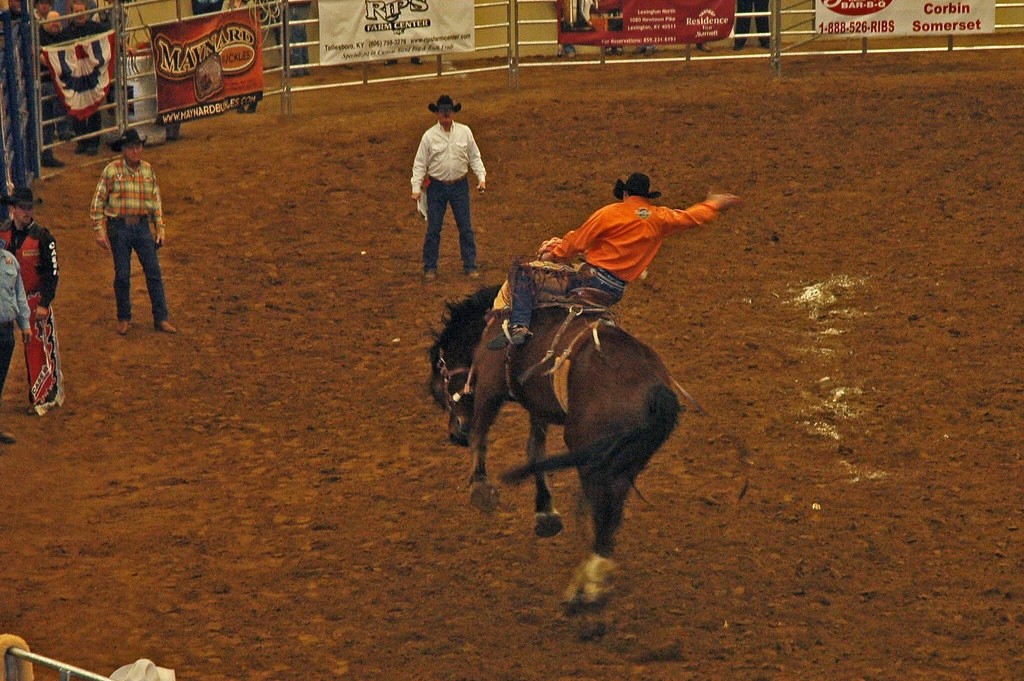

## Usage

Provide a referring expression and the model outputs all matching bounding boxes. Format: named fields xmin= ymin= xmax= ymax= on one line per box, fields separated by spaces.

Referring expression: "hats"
xmin=610 ymin=172 xmax=661 ymax=200
xmin=32 ymin=0 xmax=53 ymax=8
xmin=428 ymin=92 xmax=464 ymax=113
xmin=1 ymin=185 xmax=42 ymax=211
xmin=109 ymin=128 xmax=148 ymax=152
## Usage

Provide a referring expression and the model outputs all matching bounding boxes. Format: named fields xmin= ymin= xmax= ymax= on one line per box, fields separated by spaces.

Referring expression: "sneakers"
xmin=117 ymin=318 xmax=130 ymax=334
xmin=154 ymin=319 xmax=177 ymax=333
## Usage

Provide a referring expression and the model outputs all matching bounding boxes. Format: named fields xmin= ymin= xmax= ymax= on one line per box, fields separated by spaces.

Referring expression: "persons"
xmin=384 ymin=55 xmax=423 ymax=67
xmin=1 ymin=0 xmax=41 ymax=126
xmin=90 ymin=127 xmax=178 ymax=335
xmin=605 ymin=45 xmax=624 ymax=57
xmin=564 ymin=44 xmax=577 ymax=58
xmin=410 ymin=94 xmax=487 ymax=282
xmin=28 ymin=0 xmax=69 ymax=143
xmin=286 ymin=0 xmax=311 ymax=78
xmin=269 ymin=0 xmax=288 ymax=76
xmin=343 ymin=64 xmax=354 ymax=69
xmin=61 ymin=2 xmax=109 ymax=158
xmin=0 ymin=185 xmax=60 ymax=416
xmin=732 ymin=0 xmax=772 ymax=50
xmin=691 ymin=43 xmax=711 ymax=52
xmin=99 ymin=1 xmax=137 ymax=114
xmin=0 ymin=239 xmax=34 ymax=444
xmin=485 ymin=172 xmax=743 ymax=356
xmin=631 ymin=45 xmax=658 ymax=58
xmin=33 ymin=11 xmax=77 ymax=168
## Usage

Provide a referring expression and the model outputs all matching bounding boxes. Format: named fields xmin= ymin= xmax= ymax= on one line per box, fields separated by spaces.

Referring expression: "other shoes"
xmin=0 ymin=429 xmax=17 ymax=445
xmin=75 ymin=139 xmax=87 ymax=155
xmin=732 ymin=40 xmax=772 ymax=52
xmin=424 ymin=266 xmax=438 ymax=282
xmin=602 ymin=45 xmax=627 ymax=57
xmin=285 ymin=66 xmax=311 ymax=79
xmin=382 ymin=56 xmax=400 ymax=67
xmin=694 ymin=38 xmax=713 ymax=51
xmin=84 ymin=142 xmax=101 ymax=155
xmin=463 ymin=266 xmax=482 ymax=278
xmin=631 ymin=41 xmax=658 ymax=56
xmin=410 ymin=56 xmax=425 ymax=66
xmin=487 ymin=327 xmax=529 ymax=352
xmin=40 ymin=157 xmax=66 ymax=167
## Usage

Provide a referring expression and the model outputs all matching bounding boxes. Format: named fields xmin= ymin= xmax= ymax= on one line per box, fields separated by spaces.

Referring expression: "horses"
xmin=426 ymin=285 xmax=681 ymax=615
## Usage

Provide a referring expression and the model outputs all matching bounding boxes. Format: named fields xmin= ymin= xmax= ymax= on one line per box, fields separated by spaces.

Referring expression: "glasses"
xmin=437 ymin=108 xmax=455 ymax=114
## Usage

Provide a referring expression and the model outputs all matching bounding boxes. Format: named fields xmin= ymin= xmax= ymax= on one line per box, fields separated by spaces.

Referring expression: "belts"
xmin=434 ymin=176 xmax=467 ymax=185
xmin=110 ymin=215 xmax=153 ymax=227
